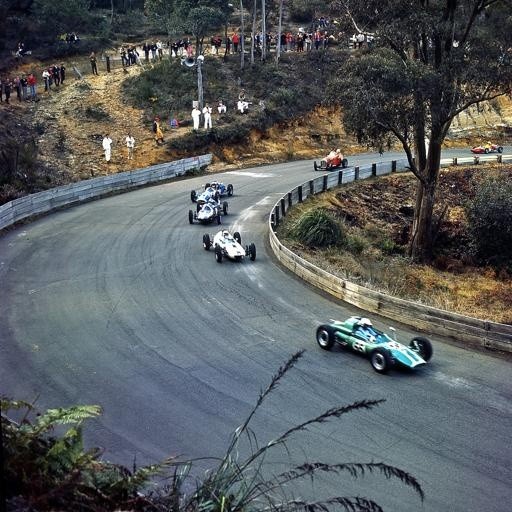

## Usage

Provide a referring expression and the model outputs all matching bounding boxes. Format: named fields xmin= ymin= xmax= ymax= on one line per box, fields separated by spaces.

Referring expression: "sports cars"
xmin=315 ymin=314 xmax=432 ymax=375
xmin=469 ymin=142 xmax=503 ymax=154
xmin=312 ymin=150 xmax=348 ymax=172
xmin=188 ymin=181 xmax=256 ymax=264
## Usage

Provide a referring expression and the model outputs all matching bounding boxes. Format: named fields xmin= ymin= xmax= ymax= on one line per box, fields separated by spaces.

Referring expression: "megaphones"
xmin=185 ymin=57 xmax=196 ymax=67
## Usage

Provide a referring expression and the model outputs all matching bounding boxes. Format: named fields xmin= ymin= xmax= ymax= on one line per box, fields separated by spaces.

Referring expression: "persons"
xmin=202 ymin=102 xmax=213 ymax=129
xmin=143 ymin=39 xmax=163 ymax=59
xmin=219 ymin=231 xmax=234 ymax=244
xmin=216 ymin=97 xmax=227 ymax=114
xmin=211 ymin=183 xmax=216 ymax=190
xmin=485 ymin=141 xmax=491 ymax=149
xmin=168 ymin=37 xmax=193 ymax=57
xmin=152 ymin=117 xmax=165 ymax=147
xmin=238 ymin=89 xmax=249 ymax=113
xmin=125 ymin=132 xmax=136 ymax=160
xmin=89 ymin=51 xmax=98 ymax=75
xmin=352 ymin=31 xmax=374 ymax=49
xmin=102 ymin=133 xmax=113 ymax=164
xmin=255 ymin=30 xmax=329 ymax=53
xmin=43 ymin=62 xmax=66 ymax=92
xmin=120 ymin=45 xmax=139 ymax=66
xmin=357 ymin=318 xmax=381 ymax=343
xmin=211 ymin=32 xmax=242 ymax=55
xmin=318 ymin=16 xmax=328 ymax=28
xmin=66 ymin=31 xmax=80 ymax=44
xmin=190 ymin=105 xmax=201 ymax=131
xmin=4 ymin=72 xmax=37 ymax=103
xmin=105 ymin=51 xmax=111 ymax=72
